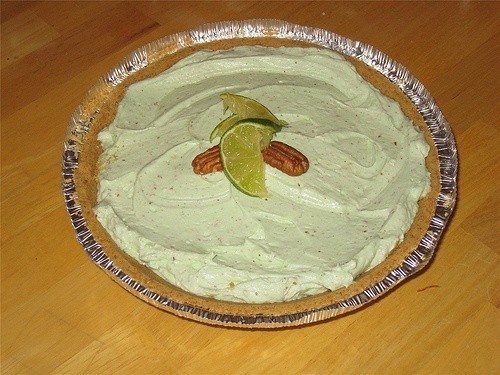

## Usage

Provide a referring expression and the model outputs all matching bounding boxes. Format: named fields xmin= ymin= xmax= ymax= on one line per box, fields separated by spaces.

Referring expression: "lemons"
xmin=210 ymin=93 xmax=289 ymax=199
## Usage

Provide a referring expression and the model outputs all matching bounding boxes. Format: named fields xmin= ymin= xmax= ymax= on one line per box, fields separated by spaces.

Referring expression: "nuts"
xmin=262 ymin=141 xmax=309 ymax=176
xmin=191 ymin=143 xmax=222 ymax=175
